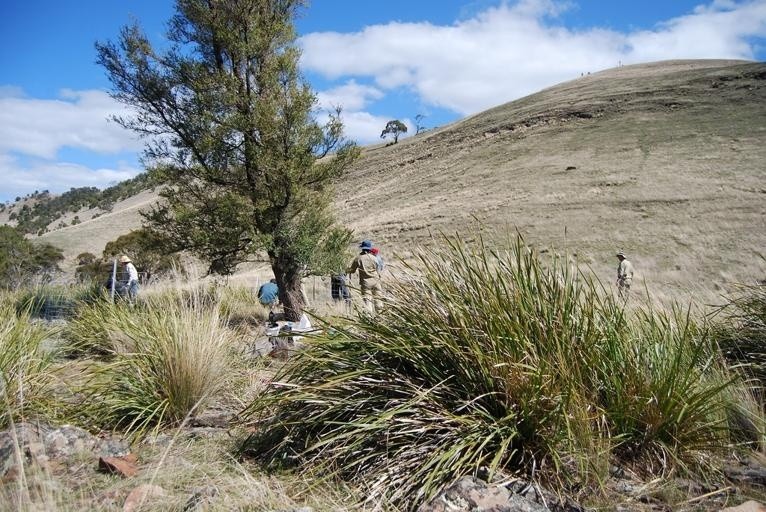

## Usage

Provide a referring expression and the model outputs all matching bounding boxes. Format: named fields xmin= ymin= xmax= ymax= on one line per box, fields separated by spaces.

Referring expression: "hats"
xmin=370 ymin=249 xmax=378 ymax=255
xmin=118 ymin=255 xmax=133 ymax=263
xmin=616 ymin=251 xmax=626 ymax=259
xmin=359 ymin=239 xmax=373 ymax=250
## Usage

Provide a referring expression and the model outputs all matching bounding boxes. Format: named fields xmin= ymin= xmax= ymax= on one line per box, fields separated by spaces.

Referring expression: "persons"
xmin=330 ymin=271 xmax=350 ymax=304
xmin=105 ymin=270 xmax=125 ymax=304
xmin=615 ymin=250 xmax=634 ymax=302
xmin=257 ymin=278 xmax=283 ymax=309
xmin=347 ymin=239 xmax=383 ymax=313
xmin=296 ymin=278 xmax=310 ymax=307
xmin=370 ymin=248 xmax=384 ymax=272
xmin=118 ymin=254 xmax=138 ymax=303
